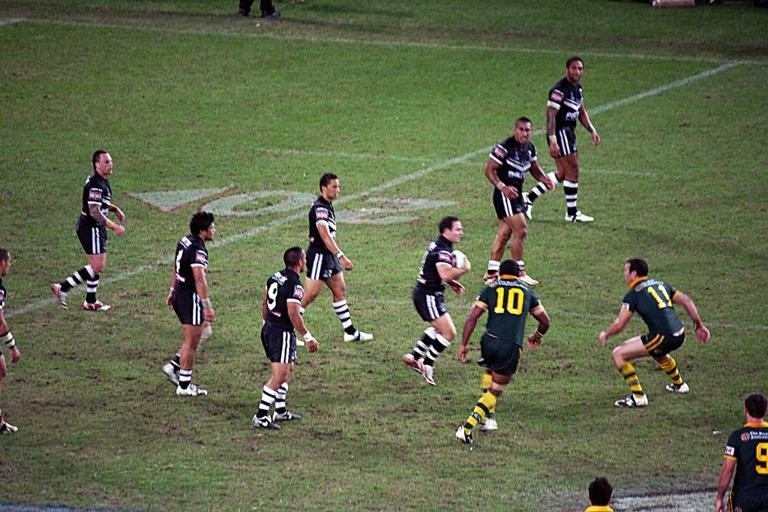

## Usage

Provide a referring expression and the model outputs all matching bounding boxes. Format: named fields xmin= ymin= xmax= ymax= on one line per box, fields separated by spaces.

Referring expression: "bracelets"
xmin=303 ymin=331 xmax=314 ymax=343
xmin=0 ymin=331 xmax=16 ymax=349
xmin=202 ymin=298 xmax=212 ymax=309
xmin=168 ymin=286 xmax=176 ymax=294
xmin=548 ymin=135 xmax=557 ymax=145
xmin=106 ymin=220 xmax=116 ymax=230
xmin=497 ymin=181 xmax=505 ymax=192
xmin=334 ymin=250 xmax=343 ymax=258
xmin=533 ymin=329 xmax=543 ymax=339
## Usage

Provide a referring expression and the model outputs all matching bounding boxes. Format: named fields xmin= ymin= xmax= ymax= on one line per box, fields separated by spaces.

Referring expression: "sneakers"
xmin=0 ymin=415 xmax=19 ymax=434
xmin=51 ymin=283 xmax=68 ymax=310
xmin=522 ymin=192 xmax=534 ymax=220
xmin=273 ymin=411 xmax=301 ymax=421
xmin=666 ymin=382 xmax=689 ymax=394
xmin=456 ymin=418 xmax=498 ymax=443
xmin=565 ymin=210 xmax=594 ymax=222
xmin=83 ymin=300 xmax=111 ymax=311
xmin=162 ymin=362 xmax=207 ymax=396
xmin=344 ymin=330 xmax=373 ymax=341
xmin=483 ymin=269 xmax=539 ymax=286
xmin=614 ymin=394 xmax=648 ymax=406
xmin=252 ymin=414 xmax=281 ymax=430
xmin=403 ymin=354 xmax=437 ymax=386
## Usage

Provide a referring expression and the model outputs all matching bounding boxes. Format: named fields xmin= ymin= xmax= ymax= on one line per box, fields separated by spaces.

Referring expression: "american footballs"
xmin=450 ymin=249 xmax=466 ymax=269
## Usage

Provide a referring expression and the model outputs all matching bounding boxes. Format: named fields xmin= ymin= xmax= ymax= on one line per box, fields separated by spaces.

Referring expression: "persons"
xmin=161 ymin=211 xmax=216 ymax=396
xmin=1 ymin=247 xmax=21 ymax=434
xmin=252 ymin=247 xmax=319 ymax=430
xmin=52 ymin=151 xmax=126 ymax=311
xmin=455 ymin=258 xmax=550 ymax=443
xmin=403 ymin=216 xmax=472 ymax=386
xmin=600 ymin=259 xmax=713 ymax=407
xmin=299 ymin=173 xmax=372 ymax=343
xmin=716 ymin=392 xmax=768 ymax=512
xmin=584 ymin=477 xmax=614 ymax=512
xmin=482 ymin=118 xmax=555 ymax=285
xmin=521 ymin=57 xmax=600 ymax=222
xmin=240 ymin=1 xmax=280 ymax=20
